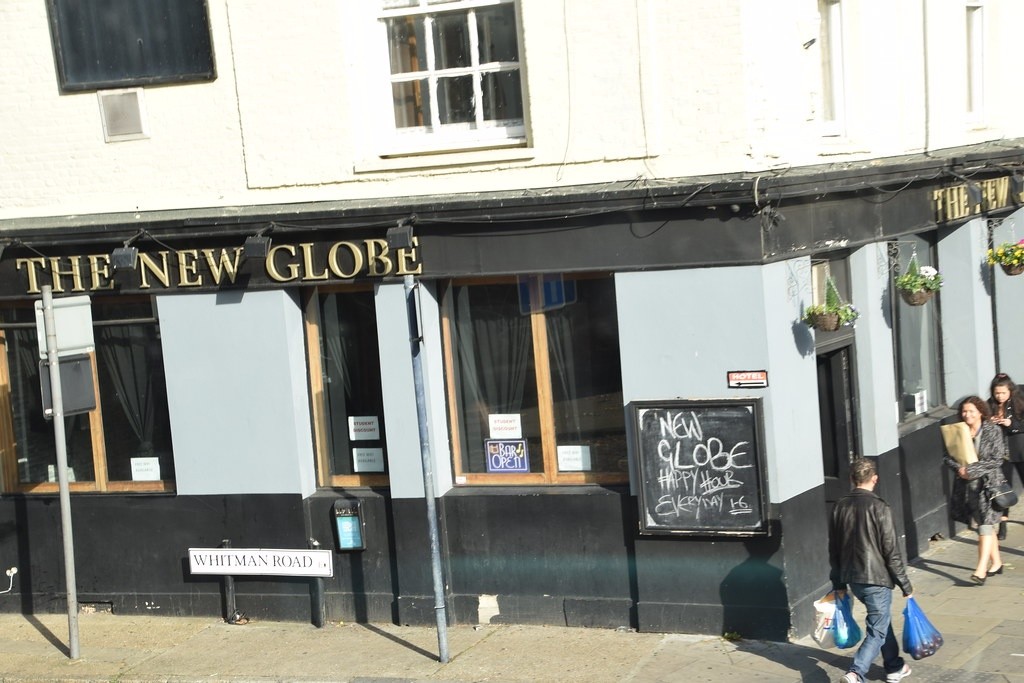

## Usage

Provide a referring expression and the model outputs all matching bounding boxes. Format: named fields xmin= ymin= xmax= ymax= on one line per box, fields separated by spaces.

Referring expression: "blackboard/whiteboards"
xmin=629 ymin=396 xmax=773 ymax=538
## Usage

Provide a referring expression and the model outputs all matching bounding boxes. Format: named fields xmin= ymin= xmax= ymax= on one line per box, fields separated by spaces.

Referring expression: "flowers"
xmin=803 ymin=304 xmax=861 ymax=330
xmin=986 ymin=239 xmax=1024 ymax=272
xmin=896 ymin=259 xmax=945 ymax=296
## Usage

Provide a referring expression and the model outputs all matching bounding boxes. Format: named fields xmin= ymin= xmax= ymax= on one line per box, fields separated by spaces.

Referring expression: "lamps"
xmin=987 ymin=162 xmax=1024 ymax=194
xmin=387 ymin=214 xmax=419 ymax=250
xmin=942 ymin=168 xmax=984 ymax=209
xmin=111 ymin=228 xmax=146 ymax=271
xmin=243 ymin=222 xmax=277 ymax=257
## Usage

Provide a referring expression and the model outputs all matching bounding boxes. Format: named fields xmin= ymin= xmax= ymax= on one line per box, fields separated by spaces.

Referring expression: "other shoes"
xmin=970 ymin=573 xmax=987 ymax=585
xmin=986 ymin=565 xmax=1004 ymax=577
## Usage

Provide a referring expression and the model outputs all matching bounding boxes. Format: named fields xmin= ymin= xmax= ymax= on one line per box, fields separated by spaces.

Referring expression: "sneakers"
xmin=840 ymin=671 xmax=860 ymax=683
xmin=886 ymin=664 xmax=912 ymax=683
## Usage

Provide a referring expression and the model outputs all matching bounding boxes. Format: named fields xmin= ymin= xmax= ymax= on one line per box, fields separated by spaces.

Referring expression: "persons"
xmin=829 ymin=458 xmax=914 ymax=683
xmin=986 ymin=373 xmax=1024 ymax=540
xmin=943 ymin=395 xmax=1007 ymax=586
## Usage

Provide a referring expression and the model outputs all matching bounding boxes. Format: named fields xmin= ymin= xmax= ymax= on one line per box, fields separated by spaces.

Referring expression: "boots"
xmin=996 ymin=516 xmax=1008 ymax=540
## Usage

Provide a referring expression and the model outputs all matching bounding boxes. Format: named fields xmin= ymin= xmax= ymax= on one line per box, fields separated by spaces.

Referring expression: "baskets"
xmin=899 ymin=288 xmax=934 ymax=306
xmin=814 ymin=311 xmax=841 ymax=331
xmin=999 ymin=261 xmax=1024 ymax=276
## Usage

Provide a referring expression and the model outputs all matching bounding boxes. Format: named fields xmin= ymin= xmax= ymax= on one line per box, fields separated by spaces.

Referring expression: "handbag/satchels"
xmin=984 ymin=479 xmax=1019 ymax=513
xmin=903 ymin=597 xmax=944 ymax=660
xmin=811 ymin=588 xmax=862 ymax=649
